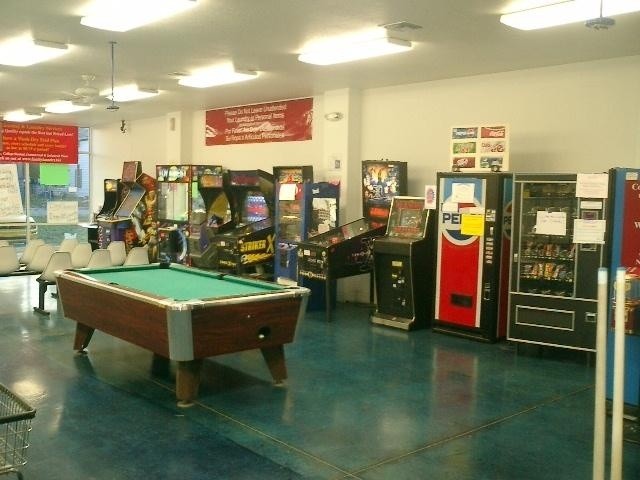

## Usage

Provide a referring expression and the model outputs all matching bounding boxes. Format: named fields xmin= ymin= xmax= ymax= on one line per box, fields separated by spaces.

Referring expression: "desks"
xmin=54 ymin=262 xmax=312 ymax=408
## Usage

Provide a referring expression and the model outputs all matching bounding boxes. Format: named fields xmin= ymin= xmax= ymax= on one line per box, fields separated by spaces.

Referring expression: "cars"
xmin=0 ymin=215 xmax=38 ymax=244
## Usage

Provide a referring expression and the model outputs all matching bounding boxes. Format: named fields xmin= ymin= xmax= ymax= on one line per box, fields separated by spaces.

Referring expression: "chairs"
xmin=0 ymin=238 xmax=149 ymax=316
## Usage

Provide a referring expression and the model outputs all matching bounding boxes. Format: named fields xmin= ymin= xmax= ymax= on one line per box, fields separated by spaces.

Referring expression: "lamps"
xmin=500 ymin=1 xmax=640 ymax=31
xmin=80 ymin=0 xmax=197 ymax=33
xmin=169 ymin=60 xmax=257 ymax=88
xmin=0 ymin=37 xmax=68 ymax=67
xmin=296 ymin=21 xmax=422 ymax=66
xmin=5 ymin=82 xmax=159 ymax=123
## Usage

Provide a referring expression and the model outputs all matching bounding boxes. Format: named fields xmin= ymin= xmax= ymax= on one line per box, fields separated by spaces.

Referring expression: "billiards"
xmin=258 ymin=334 xmax=264 ymax=339
